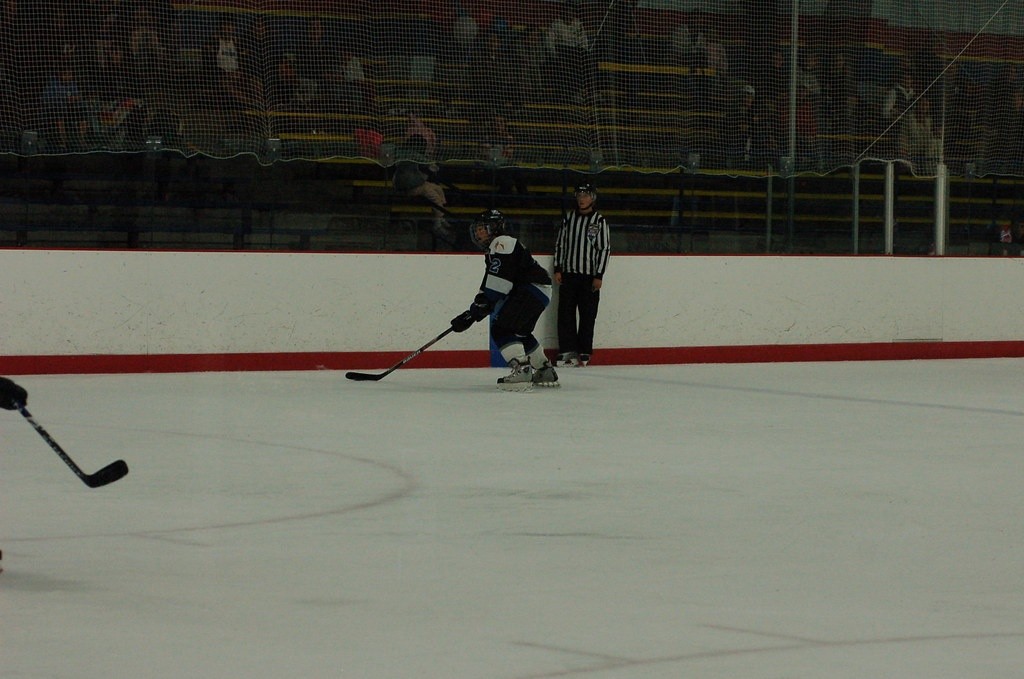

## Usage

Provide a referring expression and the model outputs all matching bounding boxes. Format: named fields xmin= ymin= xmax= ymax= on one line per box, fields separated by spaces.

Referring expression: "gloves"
xmin=450 ymin=309 xmax=477 ymax=333
xmin=0 ymin=377 xmax=27 ymax=411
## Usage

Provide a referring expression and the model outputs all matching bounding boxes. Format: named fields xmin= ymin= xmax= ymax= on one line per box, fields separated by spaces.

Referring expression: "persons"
xmin=554 ymin=180 xmax=610 ymax=367
xmin=0 ymin=3 xmax=980 ymax=227
xmin=1 ymin=376 xmax=27 ymax=410
xmin=451 ymin=210 xmax=562 ymax=393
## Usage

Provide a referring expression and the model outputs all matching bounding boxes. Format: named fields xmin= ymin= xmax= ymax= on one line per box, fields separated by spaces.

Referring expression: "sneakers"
xmin=579 ymin=353 xmax=592 ymax=368
xmin=494 ymin=356 xmax=536 ymax=393
xmin=531 ymin=361 xmax=559 ymax=388
xmin=550 ymin=351 xmax=580 ymax=368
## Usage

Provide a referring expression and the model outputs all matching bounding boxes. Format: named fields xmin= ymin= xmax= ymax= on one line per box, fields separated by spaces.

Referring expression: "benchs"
xmin=172 ymin=55 xmax=1024 ymax=245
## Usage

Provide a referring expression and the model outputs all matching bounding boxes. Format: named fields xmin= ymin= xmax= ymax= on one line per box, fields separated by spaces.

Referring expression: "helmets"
xmin=474 ymin=208 xmax=513 ymax=236
xmin=575 ymin=177 xmax=598 ymax=195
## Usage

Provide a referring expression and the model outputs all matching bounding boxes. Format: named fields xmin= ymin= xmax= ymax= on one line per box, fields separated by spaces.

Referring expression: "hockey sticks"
xmin=345 ymin=327 xmax=454 ymax=381
xmin=12 ymin=398 xmax=130 ymax=488
